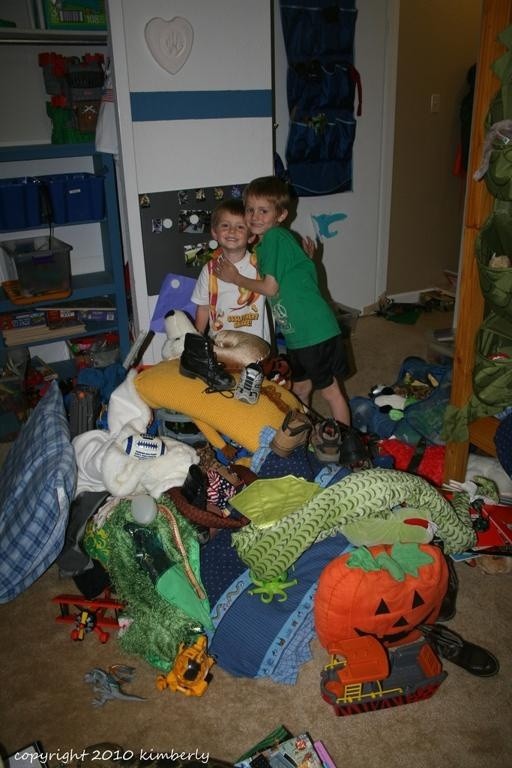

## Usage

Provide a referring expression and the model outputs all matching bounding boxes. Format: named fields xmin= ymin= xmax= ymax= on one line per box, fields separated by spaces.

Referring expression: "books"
xmin=2 ymin=297 xmax=122 ymax=350
xmin=449 ymin=505 xmax=511 ymax=563
xmin=1 ymin=354 xmax=59 ymax=388
xmin=232 ymin=724 xmax=338 ymax=768
xmin=5 ymin=741 xmax=49 ymax=768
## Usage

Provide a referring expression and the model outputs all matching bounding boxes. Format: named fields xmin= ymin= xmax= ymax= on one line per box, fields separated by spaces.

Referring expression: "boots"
xmin=437 ymin=555 xmax=458 ymax=622
xmin=416 ymin=623 xmax=500 ymax=677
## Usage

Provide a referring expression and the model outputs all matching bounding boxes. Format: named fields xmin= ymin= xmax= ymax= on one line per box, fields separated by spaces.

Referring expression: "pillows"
xmin=1 ymin=378 xmax=77 ymax=604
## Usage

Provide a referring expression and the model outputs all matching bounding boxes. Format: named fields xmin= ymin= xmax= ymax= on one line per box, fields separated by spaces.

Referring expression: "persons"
xmin=188 ymin=197 xmax=316 ymax=338
xmin=209 ymin=174 xmax=356 ymax=428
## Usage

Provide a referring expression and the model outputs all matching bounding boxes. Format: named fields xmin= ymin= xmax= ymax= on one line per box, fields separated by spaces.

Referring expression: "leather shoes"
xmin=180 ymin=332 xmax=236 ymax=391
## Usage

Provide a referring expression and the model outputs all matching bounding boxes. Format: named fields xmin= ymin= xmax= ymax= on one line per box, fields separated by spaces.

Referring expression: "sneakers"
xmin=267 ymin=370 xmax=286 ymax=386
xmin=308 ymin=418 xmax=342 ymax=462
xmin=269 ymin=407 xmax=314 ymax=458
xmin=235 ymin=362 xmax=264 ymax=405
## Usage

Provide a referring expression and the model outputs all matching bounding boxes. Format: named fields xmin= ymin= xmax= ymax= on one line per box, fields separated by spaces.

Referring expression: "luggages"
xmin=420 ymin=270 xmax=458 ymax=312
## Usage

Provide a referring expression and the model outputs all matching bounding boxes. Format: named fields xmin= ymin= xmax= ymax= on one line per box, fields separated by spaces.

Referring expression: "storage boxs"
xmin=1 ymin=235 xmax=74 ymax=299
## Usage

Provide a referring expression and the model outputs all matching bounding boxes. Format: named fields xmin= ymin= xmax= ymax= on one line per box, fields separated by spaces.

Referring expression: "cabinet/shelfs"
xmin=1 ymin=143 xmax=130 ymax=365
xmin=440 ymin=0 xmax=512 ymax=494
xmin=108 ymin=1 xmax=401 ymax=369
